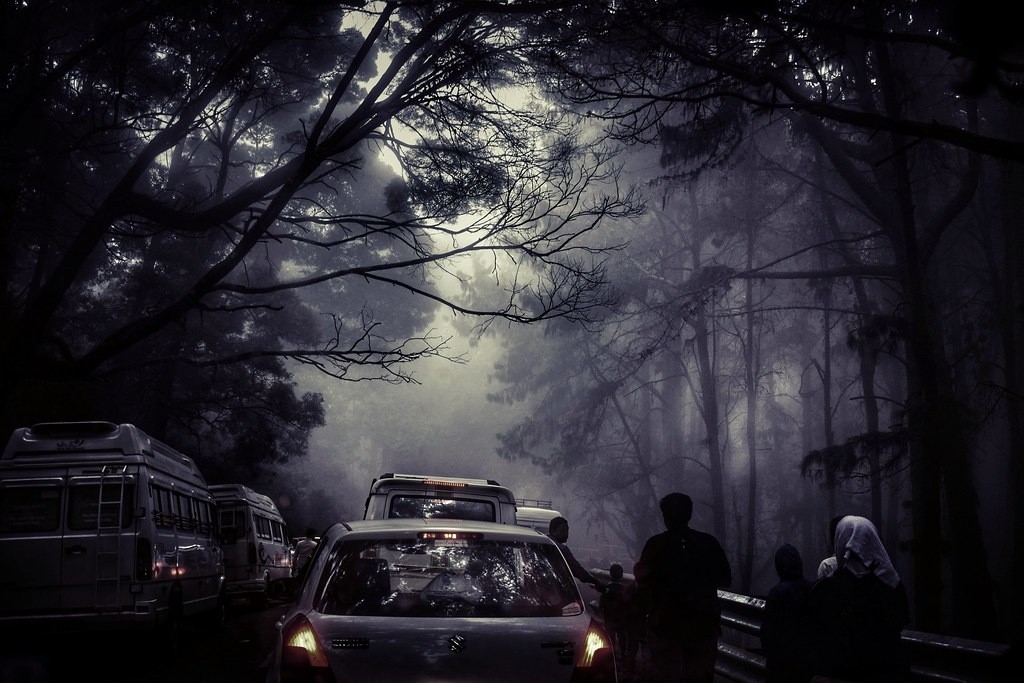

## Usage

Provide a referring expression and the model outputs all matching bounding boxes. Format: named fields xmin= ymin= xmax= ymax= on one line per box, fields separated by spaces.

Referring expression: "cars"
xmin=265 ymin=518 xmax=616 ymax=683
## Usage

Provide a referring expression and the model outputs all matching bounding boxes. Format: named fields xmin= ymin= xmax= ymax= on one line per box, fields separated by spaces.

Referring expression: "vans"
xmin=206 ymin=483 xmax=293 ymax=605
xmin=363 ymin=471 xmax=564 ymax=535
xmin=0 ymin=420 xmax=227 ymax=634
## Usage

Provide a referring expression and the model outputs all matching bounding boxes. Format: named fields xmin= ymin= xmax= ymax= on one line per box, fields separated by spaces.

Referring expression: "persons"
xmin=545 ymin=518 xmax=607 ymax=593
xmin=291 ymin=528 xmax=317 ymax=601
xmin=600 ymin=565 xmax=639 ymax=673
xmin=634 ymin=493 xmax=731 ymax=683
xmin=809 ymin=514 xmax=911 ymax=683
xmin=760 ymin=544 xmax=816 ymax=683
